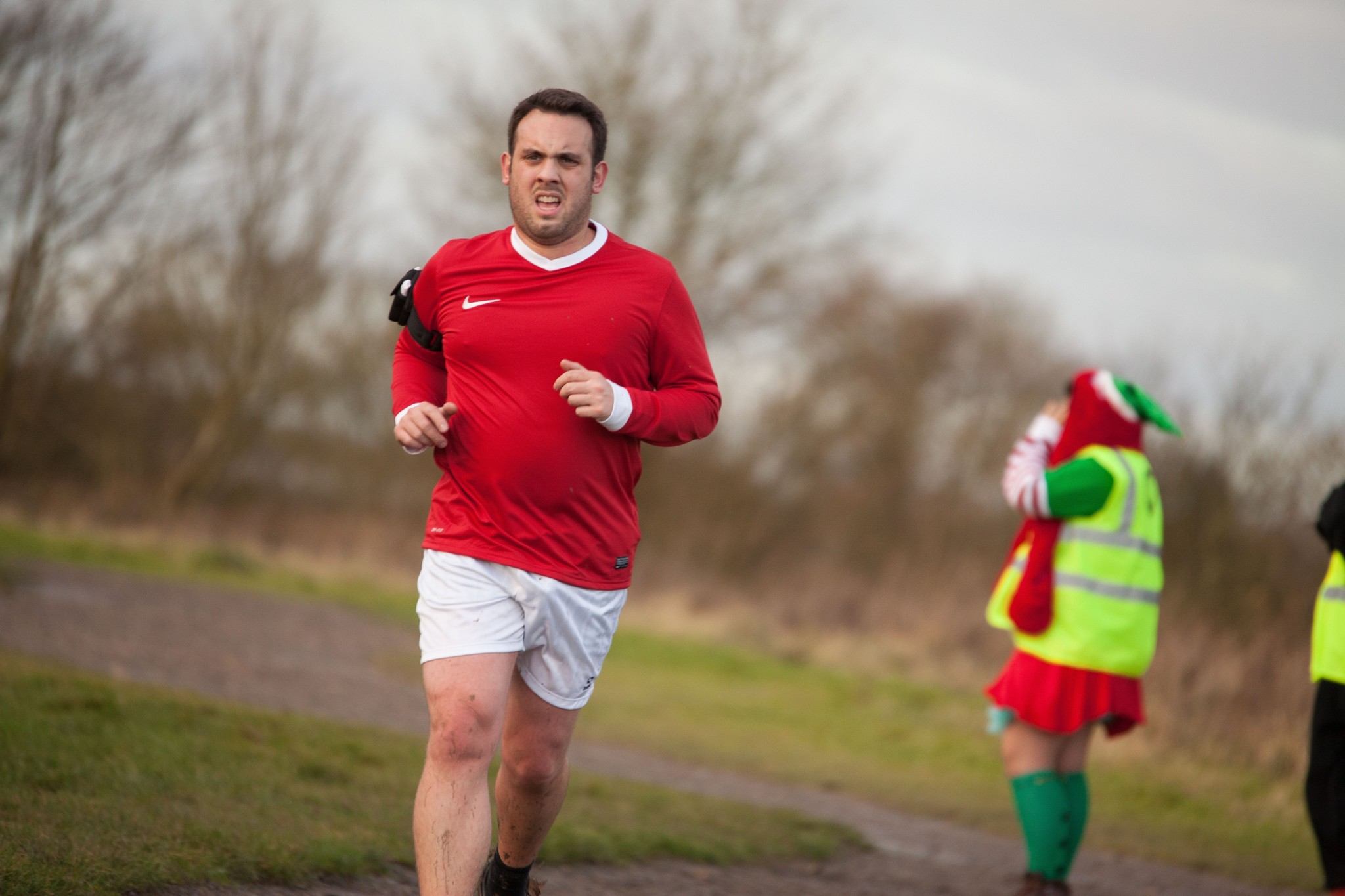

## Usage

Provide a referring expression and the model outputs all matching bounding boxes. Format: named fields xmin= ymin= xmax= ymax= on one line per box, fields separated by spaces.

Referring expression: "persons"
xmin=390 ymin=90 xmax=724 ymax=896
xmin=981 ymin=370 xmax=1183 ymax=896
xmin=1303 ymin=480 xmax=1345 ymax=896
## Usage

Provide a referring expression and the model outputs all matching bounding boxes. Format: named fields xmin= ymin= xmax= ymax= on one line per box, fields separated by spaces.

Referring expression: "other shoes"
xmin=477 ymin=850 xmax=541 ymax=896
xmin=1016 ymin=873 xmax=1065 ymax=896
